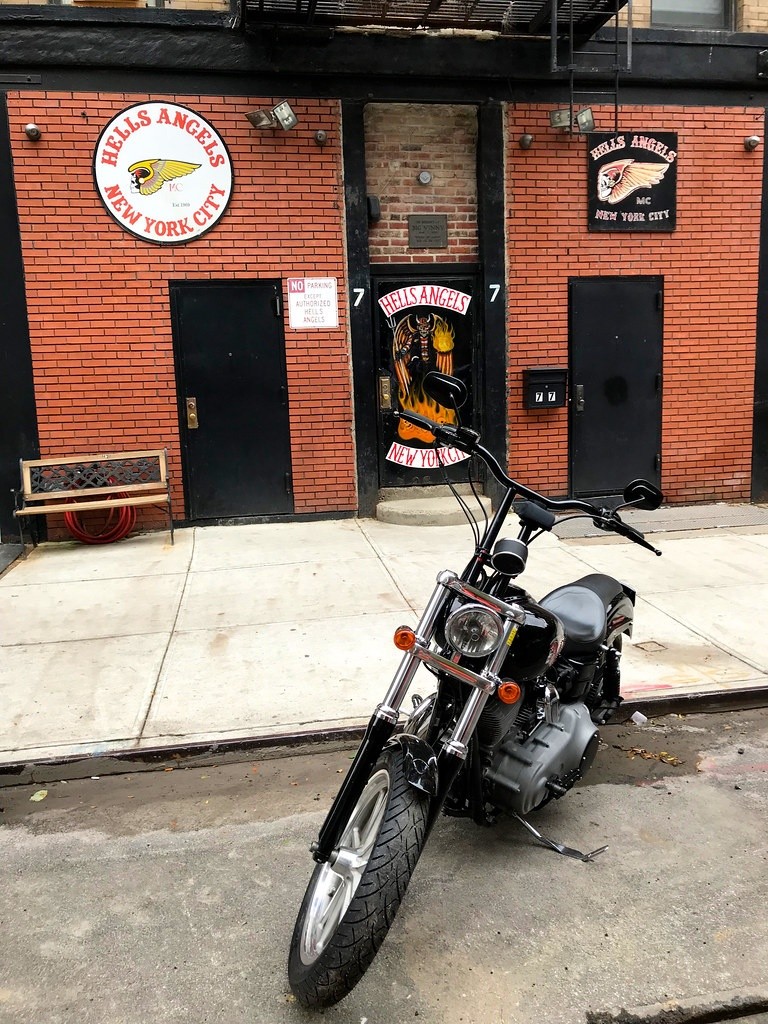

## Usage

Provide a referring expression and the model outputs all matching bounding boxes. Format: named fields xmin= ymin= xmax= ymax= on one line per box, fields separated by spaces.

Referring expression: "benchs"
xmin=10 ymin=448 xmax=174 ymax=559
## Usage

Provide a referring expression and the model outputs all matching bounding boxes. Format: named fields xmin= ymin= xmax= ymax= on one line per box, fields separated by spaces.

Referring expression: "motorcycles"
xmin=288 ymin=371 xmax=665 ymax=1009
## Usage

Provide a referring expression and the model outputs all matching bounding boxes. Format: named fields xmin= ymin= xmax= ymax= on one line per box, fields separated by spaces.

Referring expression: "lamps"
xmin=244 ymin=99 xmax=300 ymax=132
xmin=550 ymin=108 xmax=595 ymax=134
